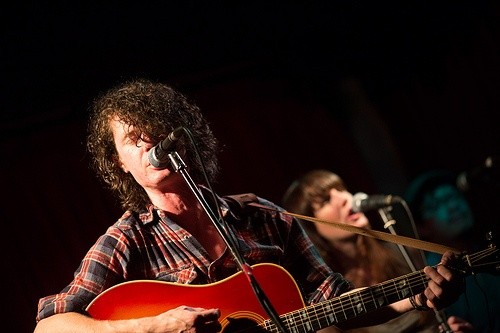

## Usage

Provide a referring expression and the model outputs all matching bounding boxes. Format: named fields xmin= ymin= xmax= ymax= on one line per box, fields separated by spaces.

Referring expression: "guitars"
xmin=85 ymin=232 xmax=500 ymax=333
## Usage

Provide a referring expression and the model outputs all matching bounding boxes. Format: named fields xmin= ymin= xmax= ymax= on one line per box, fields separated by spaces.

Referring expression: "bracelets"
xmin=408 ymin=293 xmax=432 ymax=311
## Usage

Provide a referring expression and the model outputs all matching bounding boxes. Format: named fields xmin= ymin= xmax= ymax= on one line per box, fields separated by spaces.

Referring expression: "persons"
xmin=280 ymin=169 xmax=475 ymax=333
xmin=405 ymin=174 xmax=500 ymax=333
xmin=32 ymin=81 xmax=467 ymax=333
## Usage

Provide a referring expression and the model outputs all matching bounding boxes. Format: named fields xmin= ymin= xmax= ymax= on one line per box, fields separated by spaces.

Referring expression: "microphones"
xmin=352 ymin=192 xmax=402 ymax=212
xmin=148 ymin=125 xmax=185 ymax=168
